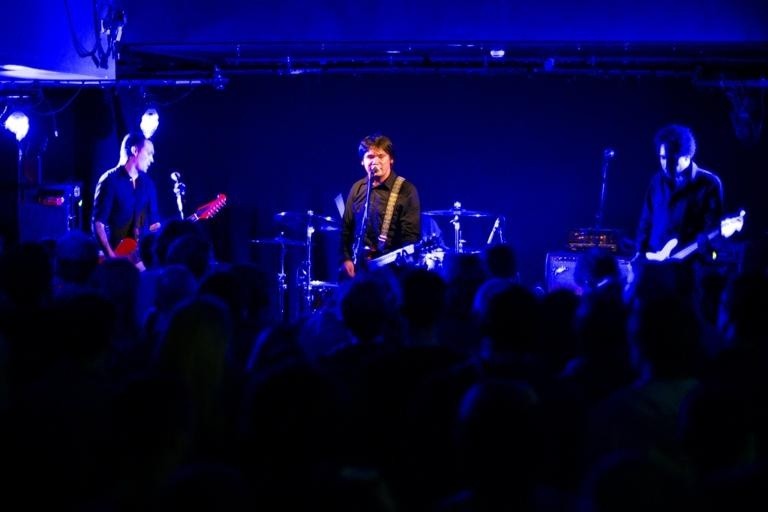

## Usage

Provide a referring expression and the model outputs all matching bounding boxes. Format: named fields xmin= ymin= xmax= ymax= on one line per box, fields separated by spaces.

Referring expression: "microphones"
xmin=369 ymin=166 xmax=379 ymax=182
xmin=170 ymin=172 xmax=186 ymax=196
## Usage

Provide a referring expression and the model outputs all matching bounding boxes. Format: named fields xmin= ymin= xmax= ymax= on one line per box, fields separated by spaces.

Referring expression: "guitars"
xmin=337 ymin=233 xmax=441 ymax=294
xmin=97 ymin=194 xmax=226 ymax=272
xmin=644 ymin=210 xmax=744 ymax=262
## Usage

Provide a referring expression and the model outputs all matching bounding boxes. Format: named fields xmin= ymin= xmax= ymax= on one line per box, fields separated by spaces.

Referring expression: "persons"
xmin=634 ymin=124 xmax=724 ymax=265
xmin=335 ymin=135 xmax=420 ymax=279
xmin=91 ymin=134 xmax=158 ymax=259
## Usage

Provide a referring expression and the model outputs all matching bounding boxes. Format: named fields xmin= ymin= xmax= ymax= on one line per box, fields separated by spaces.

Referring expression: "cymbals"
xmin=278 ymin=212 xmax=338 ymax=232
xmin=423 ymin=210 xmax=491 ymax=217
xmin=252 ymin=237 xmax=306 ymax=247
